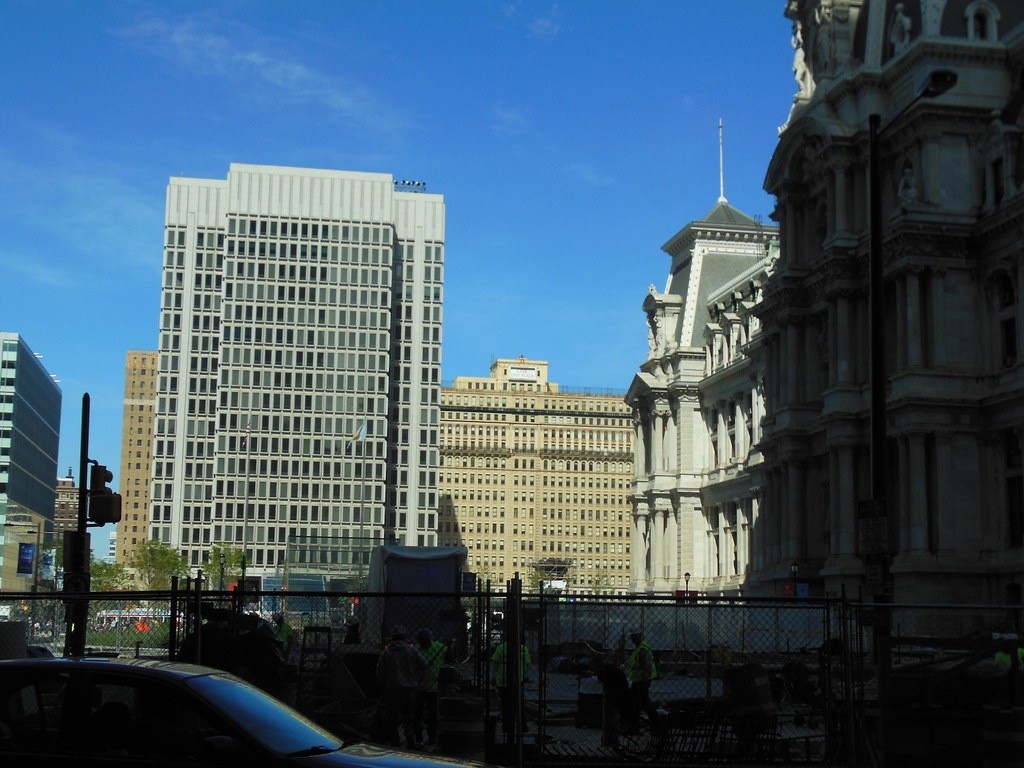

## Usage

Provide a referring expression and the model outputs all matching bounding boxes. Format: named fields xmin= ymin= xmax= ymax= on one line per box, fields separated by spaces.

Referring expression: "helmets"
xmin=389 ymin=625 xmax=407 ymax=636
xmin=272 ymin=613 xmax=282 ymax=622
xmin=626 ymin=623 xmax=643 ymax=636
xmin=345 ymin=616 xmax=360 ymax=627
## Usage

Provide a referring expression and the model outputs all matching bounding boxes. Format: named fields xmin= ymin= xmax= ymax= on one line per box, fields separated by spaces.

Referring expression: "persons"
xmin=375 ymin=627 xmax=462 ymax=749
xmin=27 ymin=621 xmax=62 ymax=636
xmin=272 ymin=613 xmax=294 ymax=654
xmin=995 ymin=647 xmax=1024 ymax=672
xmin=343 ymin=617 xmax=362 ymax=644
xmin=623 ymin=632 xmax=663 ymax=738
xmin=489 ymin=642 xmax=531 ymax=733
xmin=88 ymin=616 xmax=159 ymax=633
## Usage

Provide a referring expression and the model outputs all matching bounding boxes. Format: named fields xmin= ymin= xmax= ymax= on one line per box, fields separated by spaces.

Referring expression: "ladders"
xmin=296 ymin=625 xmax=335 ymax=709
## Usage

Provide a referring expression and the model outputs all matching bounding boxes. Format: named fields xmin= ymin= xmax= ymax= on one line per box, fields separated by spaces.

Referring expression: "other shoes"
xmin=622 ymin=729 xmax=640 ymax=736
xmin=521 ymin=726 xmax=528 ymax=733
xmin=428 ymin=737 xmax=436 ymax=744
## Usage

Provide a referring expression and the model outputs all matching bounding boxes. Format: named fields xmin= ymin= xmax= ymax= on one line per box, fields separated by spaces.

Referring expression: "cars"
xmin=0 ymin=657 xmax=502 ymax=768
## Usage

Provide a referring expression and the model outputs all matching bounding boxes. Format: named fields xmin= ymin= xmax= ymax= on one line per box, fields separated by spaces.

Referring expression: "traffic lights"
xmin=86 ymin=463 xmax=114 ymax=523
xmin=90 ymin=492 xmax=124 ymax=525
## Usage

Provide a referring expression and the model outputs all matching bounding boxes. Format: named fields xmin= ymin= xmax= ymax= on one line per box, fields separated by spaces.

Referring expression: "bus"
xmin=95 ymin=608 xmax=183 ymax=633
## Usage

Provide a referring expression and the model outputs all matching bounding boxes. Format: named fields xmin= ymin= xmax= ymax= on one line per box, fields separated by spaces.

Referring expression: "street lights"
xmin=791 ymin=560 xmax=799 ymax=599
xmin=684 ymin=572 xmax=690 ymax=627
xmin=26 ymin=529 xmax=60 ymax=642
xmin=3 ymin=521 xmax=42 ymax=639
xmin=218 ymin=553 xmax=225 ymax=609
